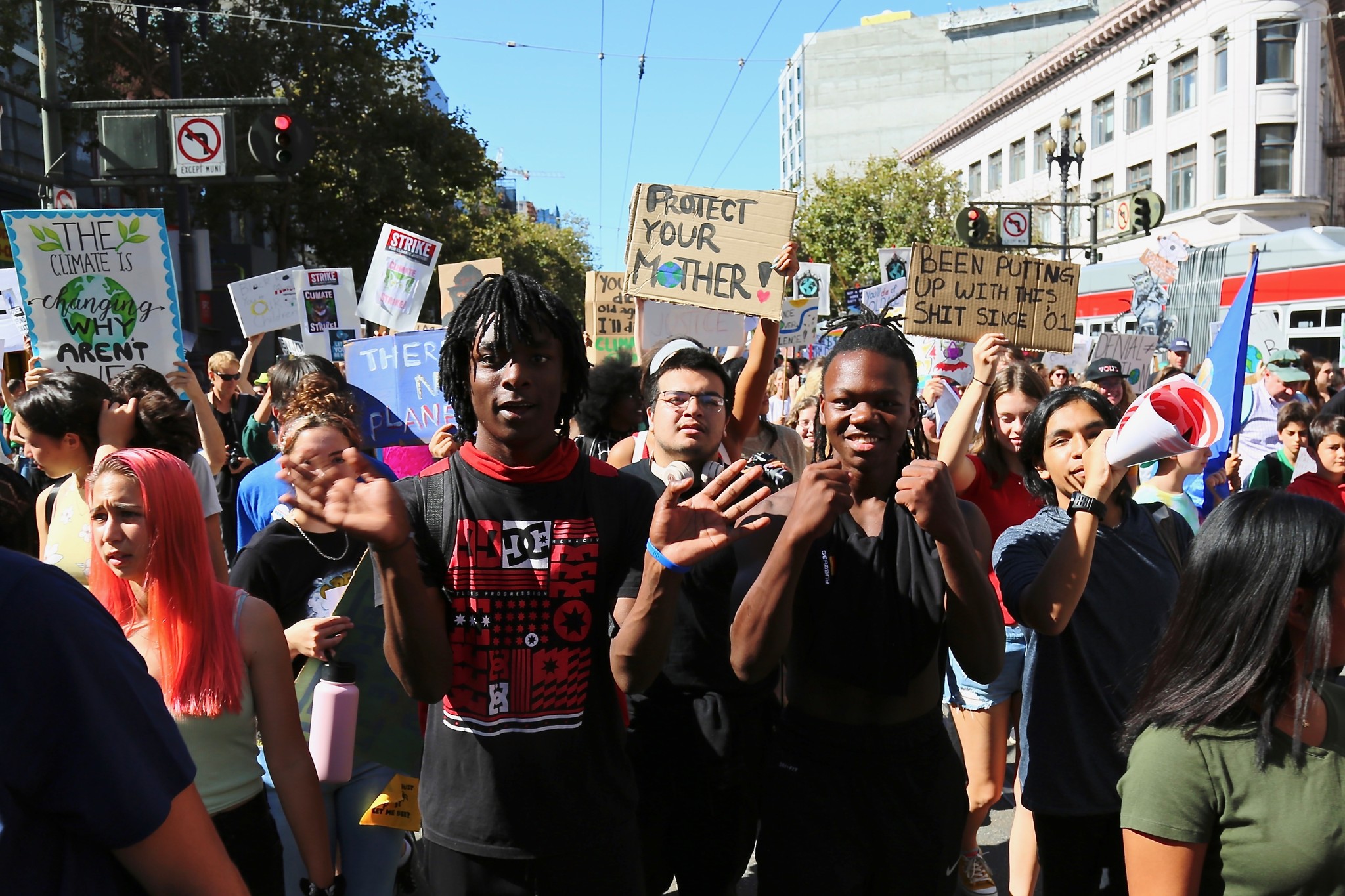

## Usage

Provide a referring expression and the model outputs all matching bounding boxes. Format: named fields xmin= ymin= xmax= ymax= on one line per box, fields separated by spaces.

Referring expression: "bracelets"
xmin=966 ymin=376 xmax=992 ymax=390
xmin=646 ymin=538 xmax=692 ymax=573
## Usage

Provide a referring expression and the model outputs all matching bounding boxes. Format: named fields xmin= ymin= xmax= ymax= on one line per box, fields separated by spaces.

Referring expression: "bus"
xmin=1074 ymin=226 xmax=1345 ymax=360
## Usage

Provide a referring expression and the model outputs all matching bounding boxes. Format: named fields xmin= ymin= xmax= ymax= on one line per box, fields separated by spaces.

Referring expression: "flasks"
xmin=309 ymin=651 xmax=359 ymax=784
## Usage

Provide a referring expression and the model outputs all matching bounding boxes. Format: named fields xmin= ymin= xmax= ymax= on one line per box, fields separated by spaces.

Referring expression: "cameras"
xmin=743 ymin=452 xmax=794 ymax=490
xmin=225 ymin=442 xmax=245 ymax=469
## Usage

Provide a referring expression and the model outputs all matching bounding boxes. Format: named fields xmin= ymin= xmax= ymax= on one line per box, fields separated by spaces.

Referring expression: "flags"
xmin=1152 ymin=248 xmax=1259 ymax=516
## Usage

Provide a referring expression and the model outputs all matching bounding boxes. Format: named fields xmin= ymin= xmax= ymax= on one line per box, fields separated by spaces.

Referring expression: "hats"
xmin=1169 ymin=337 xmax=1191 ymax=353
xmin=650 ymin=339 xmax=701 ymax=375
xmin=1267 ymin=349 xmax=1310 ymax=382
xmin=1085 ymin=358 xmax=1130 ymax=382
xmin=254 ymin=373 xmax=269 ymax=385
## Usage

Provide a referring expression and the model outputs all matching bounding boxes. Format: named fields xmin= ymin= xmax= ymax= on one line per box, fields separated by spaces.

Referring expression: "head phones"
xmin=648 ymin=450 xmax=725 ymax=487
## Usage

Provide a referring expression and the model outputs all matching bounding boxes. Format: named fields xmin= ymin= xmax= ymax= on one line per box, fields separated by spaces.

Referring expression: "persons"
xmin=0 ymin=242 xmax=1345 ymax=896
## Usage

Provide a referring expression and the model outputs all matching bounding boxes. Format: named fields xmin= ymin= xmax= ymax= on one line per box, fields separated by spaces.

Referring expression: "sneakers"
xmin=956 ymin=847 xmax=998 ymax=896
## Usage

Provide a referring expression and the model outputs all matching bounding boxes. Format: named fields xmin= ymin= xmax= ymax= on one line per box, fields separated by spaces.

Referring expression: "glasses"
xmin=215 ymin=372 xmax=241 ymax=381
xmin=650 ymin=390 xmax=728 ymax=412
xmin=1265 ymin=358 xmax=1303 ymax=368
xmin=796 ymin=420 xmax=814 ymax=429
xmin=1055 ymin=373 xmax=1067 ymax=378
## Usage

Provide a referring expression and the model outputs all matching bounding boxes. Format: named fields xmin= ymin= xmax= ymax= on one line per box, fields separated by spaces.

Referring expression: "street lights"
xmin=1043 ymin=108 xmax=1086 ymax=261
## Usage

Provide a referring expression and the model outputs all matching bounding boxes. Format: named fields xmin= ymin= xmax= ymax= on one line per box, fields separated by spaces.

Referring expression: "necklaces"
xmin=290 ymin=508 xmax=349 ymax=560
xmin=1280 ymin=712 xmax=1310 ymax=728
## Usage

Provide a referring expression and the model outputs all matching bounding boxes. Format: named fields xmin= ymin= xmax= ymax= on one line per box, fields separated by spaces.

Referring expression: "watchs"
xmin=1066 ymin=491 xmax=1107 ymax=521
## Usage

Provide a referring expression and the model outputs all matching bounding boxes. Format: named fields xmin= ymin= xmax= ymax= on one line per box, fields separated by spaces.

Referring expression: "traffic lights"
xmin=956 ymin=207 xmax=989 ymax=247
xmin=248 ymin=106 xmax=315 ymax=178
xmin=1130 ymin=190 xmax=1165 ymax=232
